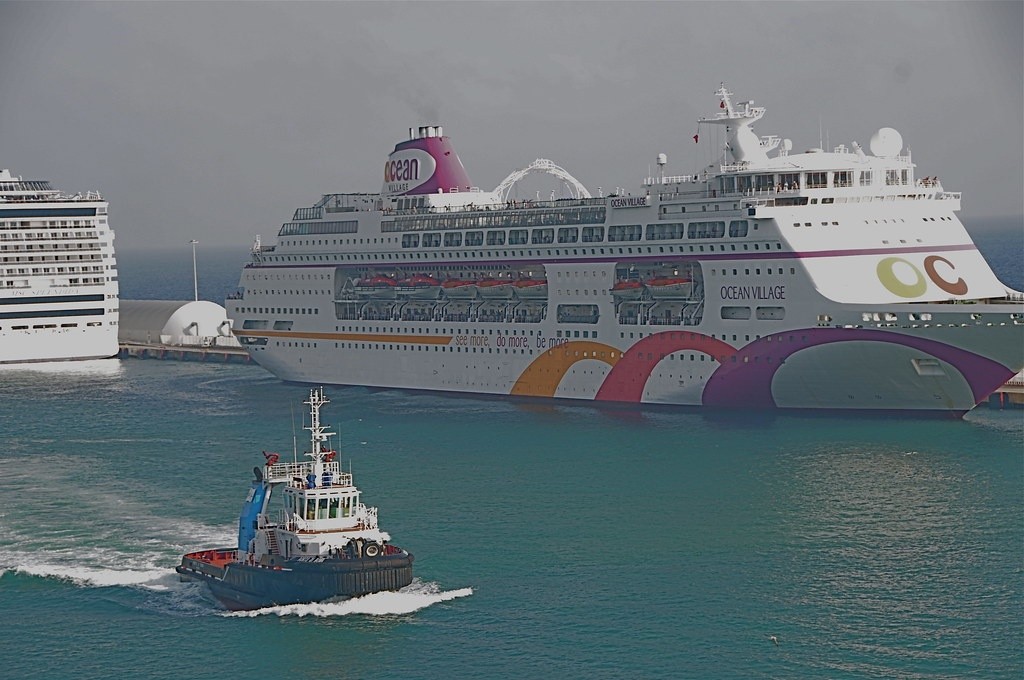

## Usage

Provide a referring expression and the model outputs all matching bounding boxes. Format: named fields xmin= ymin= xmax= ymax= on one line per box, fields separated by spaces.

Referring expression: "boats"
xmin=176 ymin=382 xmax=413 ymax=614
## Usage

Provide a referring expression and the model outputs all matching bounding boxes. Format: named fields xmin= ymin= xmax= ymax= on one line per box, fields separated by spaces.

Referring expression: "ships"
xmin=0 ymin=167 xmax=123 ymax=379
xmin=225 ymin=80 xmax=1023 ymax=415
